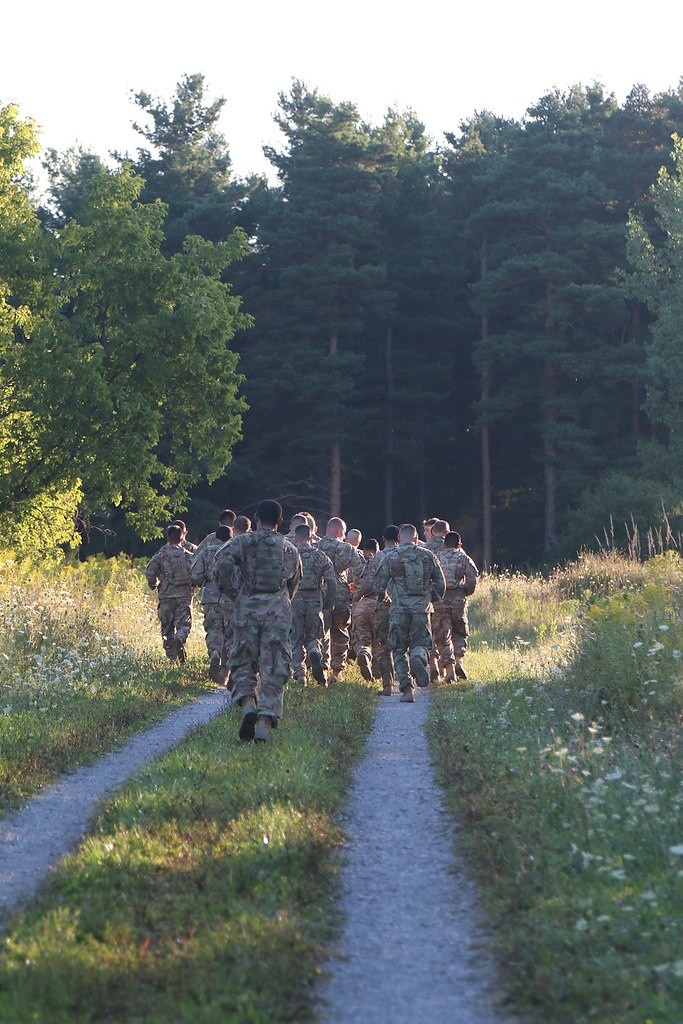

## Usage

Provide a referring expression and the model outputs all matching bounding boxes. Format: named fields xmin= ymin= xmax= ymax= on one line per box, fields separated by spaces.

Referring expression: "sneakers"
xmin=357 ymin=654 xmax=374 ymax=681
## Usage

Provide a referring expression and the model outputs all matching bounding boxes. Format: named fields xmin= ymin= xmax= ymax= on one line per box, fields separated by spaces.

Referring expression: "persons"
xmin=145 ymin=510 xmax=479 ymax=703
xmin=212 ymin=500 xmax=303 ymax=744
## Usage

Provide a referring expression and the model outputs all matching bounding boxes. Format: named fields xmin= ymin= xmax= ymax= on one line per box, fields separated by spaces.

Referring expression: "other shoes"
xmin=295 ymin=675 xmax=308 ymax=686
xmin=308 ymin=649 xmax=327 ymax=685
xmin=399 ymin=690 xmax=414 ymax=703
xmin=445 ymin=672 xmax=457 ymax=684
xmin=439 ymin=666 xmax=446 ymax=677
xmin=237 ymin=703 xmax=258 ymax=742
xmin=431 ymin=671 xmax=439 ymax=684
xmin=455 ymin=662 xmax=468 ymax=680
xmin=411 ymin=658 xmax=429 ymax=687
xmin=254 ymin=718 xmax=273 ymax=745
xmin=330 ymin=672 xmax=346 ymax=684
xmin=371 ymin=668 xmax=382 ymax=679
xmin=209 ymin=651 xmax=229 ymax=686
xmin=377 ymin=686 xmax=391 ymax=696
xmin=163 ymin=638 xmax=188 ymax=665
